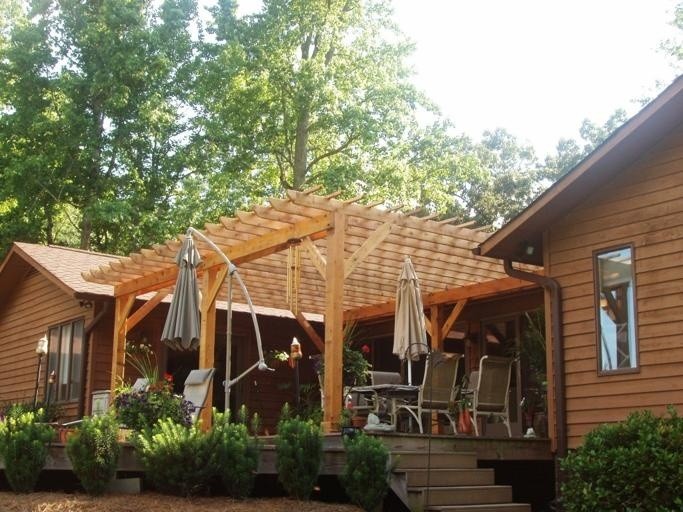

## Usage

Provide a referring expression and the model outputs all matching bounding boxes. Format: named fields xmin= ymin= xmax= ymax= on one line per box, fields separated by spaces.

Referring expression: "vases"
xmin=316 ymin=370 xmax=324 ymax=388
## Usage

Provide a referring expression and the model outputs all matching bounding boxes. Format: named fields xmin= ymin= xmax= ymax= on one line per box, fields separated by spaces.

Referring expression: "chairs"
xmin=370 ymin=353 xmax=515 ymax=438
xmin=131 ymin=366 xmax=216 ymax=426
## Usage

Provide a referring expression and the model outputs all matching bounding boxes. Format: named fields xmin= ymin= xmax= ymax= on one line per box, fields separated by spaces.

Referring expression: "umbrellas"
xmin=161 ymin=227 xmax=267 ymax=420
xmin=392 ymin=256 xmax=428 ymax=432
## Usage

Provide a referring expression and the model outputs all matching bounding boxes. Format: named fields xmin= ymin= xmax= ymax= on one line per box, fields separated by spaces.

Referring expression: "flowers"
xmin=308 ymin=312 xmax=372 ymax=388
xmin=109 ymin=337 xmax=194 ymax=431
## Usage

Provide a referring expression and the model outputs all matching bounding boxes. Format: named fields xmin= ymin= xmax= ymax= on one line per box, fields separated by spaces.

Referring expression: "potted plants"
xmin=506 ymin=303 xmax=548 ymax=438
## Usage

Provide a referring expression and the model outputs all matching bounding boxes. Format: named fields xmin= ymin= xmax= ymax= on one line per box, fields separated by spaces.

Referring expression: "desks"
xmin=350 ymin=382 xmax=472 ymax=433
